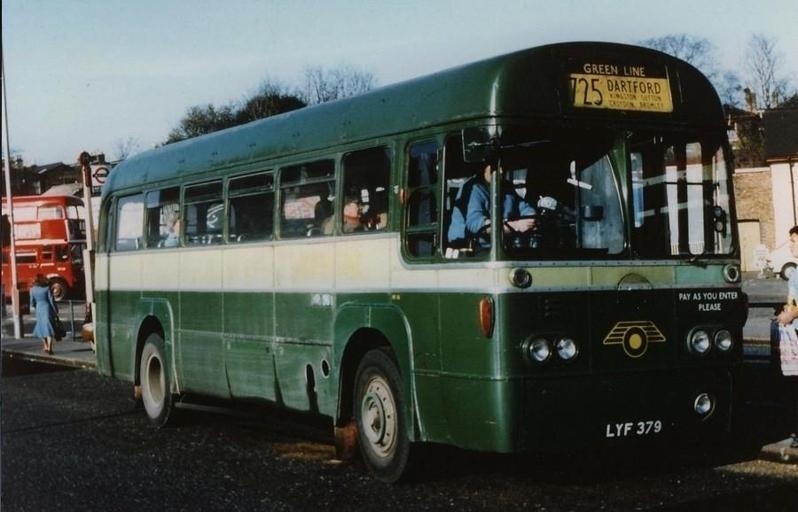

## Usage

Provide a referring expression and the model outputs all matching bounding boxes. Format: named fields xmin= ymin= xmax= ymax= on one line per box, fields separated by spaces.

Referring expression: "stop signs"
xmin=89 ymin=165 xmax=111 ymax=186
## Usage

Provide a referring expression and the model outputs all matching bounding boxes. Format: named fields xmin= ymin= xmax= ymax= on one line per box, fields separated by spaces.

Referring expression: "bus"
xmin=2 ymin=194 xmax=86 ymax=301
xmin=90 ymin=40 xmax=747 ymax=484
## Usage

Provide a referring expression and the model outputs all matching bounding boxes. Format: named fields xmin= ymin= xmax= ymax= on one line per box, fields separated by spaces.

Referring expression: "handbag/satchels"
xmin=56 ymin=319 xmax=66 ymax=338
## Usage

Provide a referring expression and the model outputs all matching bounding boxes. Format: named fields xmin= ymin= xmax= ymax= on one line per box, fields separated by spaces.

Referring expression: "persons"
xmin=164 ymin=214 xmax=180 ymax=248
xmin=29 ymin=272 xmax=59 ymax=355
xmin=312 ymin=183 xmax=388 ymax=237
xmin=445 ymin=155 xmax=536 ymax=260
xmin=69 ymin=222 xmax=85 ymax=259
xmin=767 ymin=224 xmax=798 ymax=337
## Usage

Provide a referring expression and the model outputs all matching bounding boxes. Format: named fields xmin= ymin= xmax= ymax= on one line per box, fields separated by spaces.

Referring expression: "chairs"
xmin=134 ymin=226 xmax=323 ymax=250
